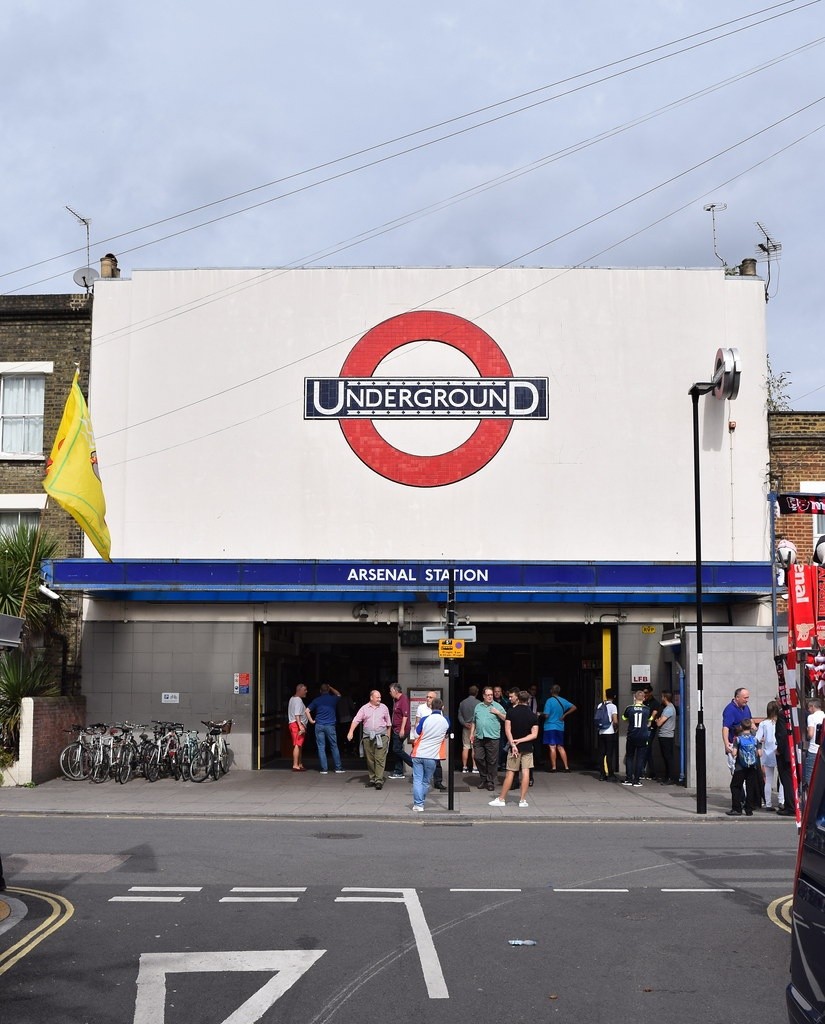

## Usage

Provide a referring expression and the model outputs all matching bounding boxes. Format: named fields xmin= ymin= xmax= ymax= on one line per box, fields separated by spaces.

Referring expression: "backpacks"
xmin=736 ymin=734 xmax=758 ymax=768
xmin=594 ymin=700 xmax=613 ymax=729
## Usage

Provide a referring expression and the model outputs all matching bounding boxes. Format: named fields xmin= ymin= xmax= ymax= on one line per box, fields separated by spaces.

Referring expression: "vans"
xmin=786 ymin=718 xmax=825 ymax=1024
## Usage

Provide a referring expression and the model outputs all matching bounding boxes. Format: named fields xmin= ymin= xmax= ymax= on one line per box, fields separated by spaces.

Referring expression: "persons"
xmin=388 ymin=683 xmax=413 ymax=779
xmin=621 ymin=691 xmax=650 ymax=787
xmin=470 ymin=687 xmax=507 ymax=791
xmin=411 ymin=698 xmax=450 ymax=811
xmin=528 ymin=685 xmax=543 ymax=716
xmin=488 ymin=690 xmax=540 ymax=808
xmin=347 ymin=690 xmax=392 ymax=790
xmin=722 ymin=688 xmax=825 ymax=816
xmin=493 ymin=687 xmax=511 ymax=772
xmin=597 ymin=688 xmax=619 ymax=782
xmin=458 ymin=686 xmax=482 ymax=774
xmin=637 ymin=685 xmax=660 ymax=780
xmin=416 ymin=692 xmax=446 ymax=789
xmin=305 ymin=684 xmax=346 ymax=774
xmin=655 ymin=690 xmax=677 ymax=785
xmin=543 ymin=685 xmax=576 ymax=773
xmin=288 ymin=684 xmax=308 ymax=772
xmin=503 ymin=687 xmax=535 ymax=790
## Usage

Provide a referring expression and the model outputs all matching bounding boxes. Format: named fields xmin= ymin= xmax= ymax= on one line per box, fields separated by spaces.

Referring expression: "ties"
xmin=530 ymin=697 xmax=534 ymax=713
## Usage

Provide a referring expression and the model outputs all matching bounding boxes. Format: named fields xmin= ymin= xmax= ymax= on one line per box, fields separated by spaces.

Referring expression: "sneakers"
xmin=462 ymin=767 xmax=471 ymax=773
xmin=488 ymin=797 xmax=505 ymax=807
xmin=472 ymin=768 xmax=479 ymax=774
xmin=519 ymin=799 xmax=528 ymax=807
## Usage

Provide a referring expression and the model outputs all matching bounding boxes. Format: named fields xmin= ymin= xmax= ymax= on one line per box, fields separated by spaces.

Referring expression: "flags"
xmin=41 ymin=367 xmax=113 ymax=564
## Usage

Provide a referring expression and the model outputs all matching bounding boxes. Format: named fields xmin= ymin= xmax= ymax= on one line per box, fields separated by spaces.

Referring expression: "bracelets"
xmin=512 ymin=745 xmax=515 ymax=747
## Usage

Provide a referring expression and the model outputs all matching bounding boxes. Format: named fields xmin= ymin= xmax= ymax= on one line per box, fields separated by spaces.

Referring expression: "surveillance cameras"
xmin=39 ymin=585 xmax=60 ymax=599
xmin=658 ymin=638 xmax=681 ymax=647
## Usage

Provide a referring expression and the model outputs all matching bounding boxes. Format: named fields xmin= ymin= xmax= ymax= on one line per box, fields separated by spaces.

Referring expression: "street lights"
xmin=688 ymin=380 xmax=717 ymax=812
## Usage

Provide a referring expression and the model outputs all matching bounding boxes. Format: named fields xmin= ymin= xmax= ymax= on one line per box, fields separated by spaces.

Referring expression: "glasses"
xmin=484 ymin=694 xmax=493 ymax=696
xmin=644 ymin=692 xmax=649 ymax=694
xmin=427 ymin=696 xmax=434 ymax=699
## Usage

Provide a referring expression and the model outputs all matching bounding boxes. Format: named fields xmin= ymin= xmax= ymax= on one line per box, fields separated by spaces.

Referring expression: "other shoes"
xmin=529 ymin=780 xmax=533 ymax=786
xmin=388 ymin=772 xmax=405 ymax=779
xmin=597 ymin=770 xmax=685 ymax=787
xmin=292 ymin=765 xmax=306 ymax=771
xmin=335 ymin=769 xmax=346 ymax=774
xmin=486 ymin=782 xmax=494 ymax=791
xmin=549 ymin=769 xmax=557 ymax=773
xmin=320 ymin=770 xmax=328 ymax=774
xmin=726 ymin=802 xmax=795 ymax=816
xmin=478 ymin=781 xmax=487 ymax=789
xmin=365 ymin=782 xmax=375 ymax=787
xmin=561 ymin=769 xmax=571 ymax=773
xmin=375 ymin=783 xmax=382 ymax=789
xmin=510 ymin=784 xmax=519 ymax=790
xmin=435 ymin=782 xmax=447 ymax=789
xmin=412 ymin=805 xmax=425 ymax=812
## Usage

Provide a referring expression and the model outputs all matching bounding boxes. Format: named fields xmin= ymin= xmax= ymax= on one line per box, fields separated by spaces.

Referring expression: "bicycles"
xmin=60 ymin=719 xmax=236 ymax=784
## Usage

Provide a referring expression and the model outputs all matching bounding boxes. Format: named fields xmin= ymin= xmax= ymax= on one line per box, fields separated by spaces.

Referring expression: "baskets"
xmin=214 ymin=720 xmax=232 ymax=733
